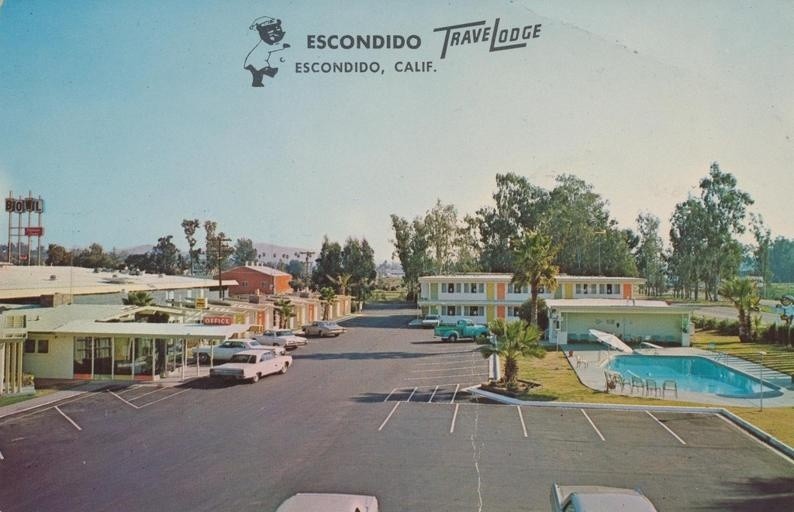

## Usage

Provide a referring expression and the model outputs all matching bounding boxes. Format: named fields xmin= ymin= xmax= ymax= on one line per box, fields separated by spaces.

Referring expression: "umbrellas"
xmin=586 ymin=327 xmax=635 ymax=371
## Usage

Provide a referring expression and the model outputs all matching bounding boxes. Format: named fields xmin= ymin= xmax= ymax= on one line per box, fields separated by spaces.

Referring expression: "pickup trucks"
xmin=434 ymin=320 xmax=490 ymax=342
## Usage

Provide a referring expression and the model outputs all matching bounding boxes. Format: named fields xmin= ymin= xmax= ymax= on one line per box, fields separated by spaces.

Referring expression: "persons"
xmin=551 ymin=308 xmax=560 ymax=329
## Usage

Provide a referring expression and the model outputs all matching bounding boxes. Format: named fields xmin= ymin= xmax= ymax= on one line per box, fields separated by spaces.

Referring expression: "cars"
xmin=210 ymin=349 xmax=293 ymax=383
xmin=302 ymin=321 xmax=347 ymax=337
xmin=252 ymin=329 xmax=309 ymax=348
xmin=422 ymin=314 xmax=442 ymax=326
xmin=191 ymin=340 xmax=259 ymax=363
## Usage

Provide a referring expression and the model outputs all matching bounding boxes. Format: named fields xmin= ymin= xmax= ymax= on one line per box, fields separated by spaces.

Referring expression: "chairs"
xmin=631 ymin=375 xmax=647 ymax=396
xmin=635 ymin=335 xmax=663 ymax=351
xmin=609 ymin=373 xmax=631 ymax=393
xmin=712 ymin=350 xmax=730 ymax=361
xmin=646 ymin=379 xmax=661 ymax=398
xmin=576 ymin=355 xmax=588 ymax=369
xmin=658 ymin=380 xmax=677 ymax=400
